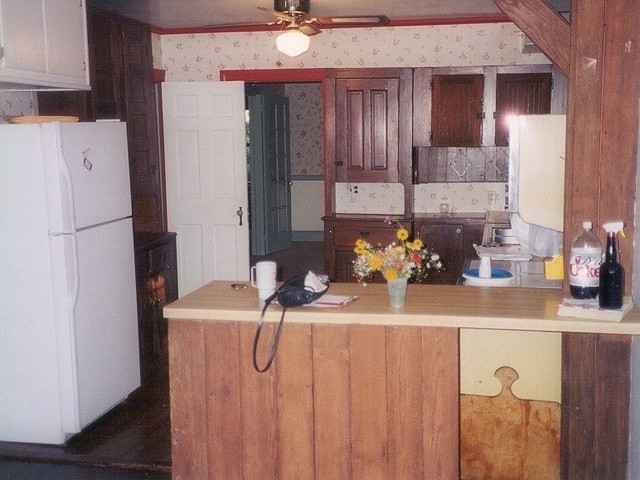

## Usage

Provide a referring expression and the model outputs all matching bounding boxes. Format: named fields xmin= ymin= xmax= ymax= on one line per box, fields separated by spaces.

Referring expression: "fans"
xmin=200 ymin=1 xmax=390 ymax=56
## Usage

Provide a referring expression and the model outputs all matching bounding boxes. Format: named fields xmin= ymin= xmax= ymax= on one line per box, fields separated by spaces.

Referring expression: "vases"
xmin=386 ymin=280 xmax=407 ymax=305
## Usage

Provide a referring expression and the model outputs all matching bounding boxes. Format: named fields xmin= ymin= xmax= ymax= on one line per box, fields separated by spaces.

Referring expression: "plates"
xmin=459 ymin=267 xmax=517 ymax=285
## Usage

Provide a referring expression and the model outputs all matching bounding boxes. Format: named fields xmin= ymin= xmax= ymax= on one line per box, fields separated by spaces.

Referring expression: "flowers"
xmin=347 ymin=218 xmax=443 ymax=282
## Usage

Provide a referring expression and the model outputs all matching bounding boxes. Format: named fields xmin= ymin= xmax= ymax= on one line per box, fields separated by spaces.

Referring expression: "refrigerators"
xmin=0 ymin=120 xmax=143 ymax=447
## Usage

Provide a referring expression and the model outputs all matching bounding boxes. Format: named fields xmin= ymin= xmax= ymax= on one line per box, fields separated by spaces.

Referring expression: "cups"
xmin=250 ymin=260 xmax=279 ymax=304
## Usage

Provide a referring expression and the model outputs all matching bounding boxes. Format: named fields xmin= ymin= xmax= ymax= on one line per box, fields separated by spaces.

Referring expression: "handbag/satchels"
xmin=253 ymin=272 xmax=330 ymax=372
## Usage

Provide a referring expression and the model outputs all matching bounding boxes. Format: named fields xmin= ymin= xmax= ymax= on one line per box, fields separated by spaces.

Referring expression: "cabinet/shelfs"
xmin=324 ymin=217 xmax=413 ymax=284
xmin=495 ymin=72 xmax=554 ymax=148
xmin=431 ymin=75 xmax=483 ymax=146
xmin=415 ymin=217 xmax=483 ymax=285
xmin=0 ymin=0 xmax=91 ymax=93
xmin=334 ymin=75 xmax=399 ymax=183
xmin=39 ymin=10 xmax=176 ymax=399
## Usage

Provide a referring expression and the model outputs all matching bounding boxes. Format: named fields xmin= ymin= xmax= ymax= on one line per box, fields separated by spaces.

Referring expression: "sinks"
xmin=491 ymin=227 xmax=513 ymax=237
xmin=493 ymin=236 xmax=519 ymax=245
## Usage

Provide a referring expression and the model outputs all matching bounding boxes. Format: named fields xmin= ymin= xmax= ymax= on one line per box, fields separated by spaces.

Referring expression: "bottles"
xmin=597 ymin=231 xmax=625 ymax=311
xmin=570 ymin=221 xmax=603 ymax=303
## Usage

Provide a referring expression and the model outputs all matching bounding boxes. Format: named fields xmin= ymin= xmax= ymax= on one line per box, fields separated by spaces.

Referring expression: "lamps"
xmin=274 ymin=28 xmax=309 ymax=55
